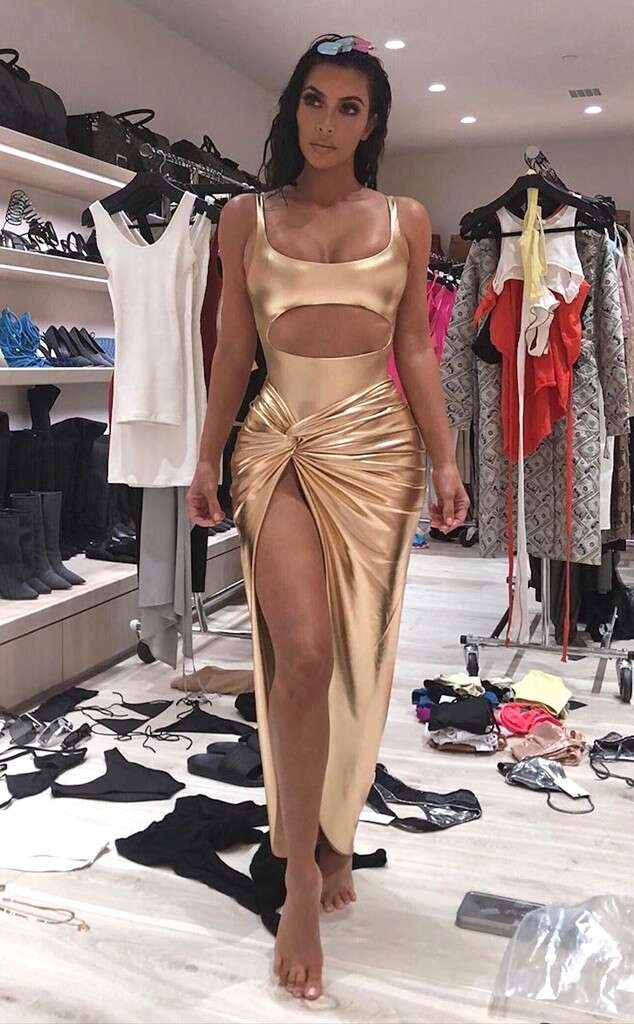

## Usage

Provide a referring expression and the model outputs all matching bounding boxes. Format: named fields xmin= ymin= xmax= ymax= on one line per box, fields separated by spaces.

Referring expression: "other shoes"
xmin=87 ymin=536 xmax=137 ymax=564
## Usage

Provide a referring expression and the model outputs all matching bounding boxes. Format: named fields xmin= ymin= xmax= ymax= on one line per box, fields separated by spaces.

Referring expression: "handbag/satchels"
xmin=68 ymin=107 xmax=175 ymax=178
xmin=172 ymin=134 xmax=248 ymax=200
xmin=0 ymin=47 xmax=68 ymax=145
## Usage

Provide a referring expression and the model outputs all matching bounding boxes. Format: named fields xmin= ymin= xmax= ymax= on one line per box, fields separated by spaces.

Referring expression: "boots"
xmin=9 ymin=492 xmax=72 ymax=589
xmin=0 ymin=512 xmax=39 ymax=600
xmin=0 ymin=385 xmax=137 ymax=562
xmin=30 ymin=490 xmax=86 ymax=585
xmin=0 ymin=506 xmax=52 ymax=595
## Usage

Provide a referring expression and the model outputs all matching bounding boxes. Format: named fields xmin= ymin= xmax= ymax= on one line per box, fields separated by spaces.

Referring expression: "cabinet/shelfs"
xmin=0 ymin=124 xmax=136 ymax=387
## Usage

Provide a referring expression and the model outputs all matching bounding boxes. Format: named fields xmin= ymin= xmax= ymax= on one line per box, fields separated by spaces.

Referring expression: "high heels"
xmin=28 ymin=218 xmax=83 ymax=259
xmin=58 ymin=325 xmax=105 ymax=366
xmin=69 ymin=327 xmax=114 ymax=366
xmin=0 ymin=305 xmax=54 ymax=367
xmin=44 ymin=325 xmax=93 ymax=366
xmin=60 ymin=232 xmax=103 ymax=263
xmin=80 ymin=328 xmax=114 ymax=364
xmin=0 ymin=189 xmax=46 ymax=253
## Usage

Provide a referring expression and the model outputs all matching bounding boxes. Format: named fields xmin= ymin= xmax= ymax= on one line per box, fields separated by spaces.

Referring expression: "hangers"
xmin=427 ymin=150 xmax=618 ymax=292
xmin=80 ymin=150 xmax=256 ymax=228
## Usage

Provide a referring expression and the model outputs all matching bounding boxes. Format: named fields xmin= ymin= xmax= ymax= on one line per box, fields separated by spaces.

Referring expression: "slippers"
xmin=187 ymin=734 xmax=264 ymax=788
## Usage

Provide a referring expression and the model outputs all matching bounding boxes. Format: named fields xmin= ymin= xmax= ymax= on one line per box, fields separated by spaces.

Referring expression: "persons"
xmin=184 ymin=33 xmax=471 ymax=1000
